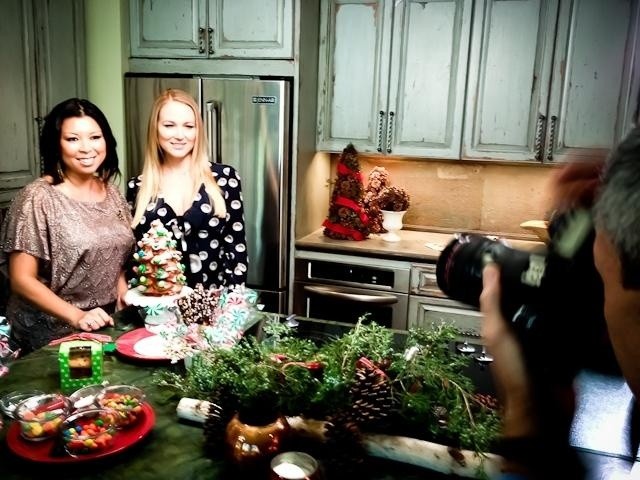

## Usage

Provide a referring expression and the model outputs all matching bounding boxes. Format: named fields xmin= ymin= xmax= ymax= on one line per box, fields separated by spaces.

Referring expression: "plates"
xmin=6 ymin=400 xmax=156 ymax=464
xmin=114 ymin=327 xmax=244 ymax=361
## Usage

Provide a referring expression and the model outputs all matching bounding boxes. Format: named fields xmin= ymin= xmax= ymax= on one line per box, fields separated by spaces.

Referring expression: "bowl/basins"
xmin=0 ymin=384 xmax=147 ymax=459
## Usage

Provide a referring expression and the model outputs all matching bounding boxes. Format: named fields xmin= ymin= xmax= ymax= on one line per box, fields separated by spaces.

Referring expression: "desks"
xmin=0 ymin=308 xmax=435 ymax=480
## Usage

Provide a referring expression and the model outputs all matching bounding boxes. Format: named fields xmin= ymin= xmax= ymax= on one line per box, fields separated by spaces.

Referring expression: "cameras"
xmin=436 ymin=208 xmax=622 ymax=389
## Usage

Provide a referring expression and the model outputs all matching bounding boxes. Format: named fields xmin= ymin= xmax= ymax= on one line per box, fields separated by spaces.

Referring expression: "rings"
xmin=89 ymin=319 xmax=97 ymax=327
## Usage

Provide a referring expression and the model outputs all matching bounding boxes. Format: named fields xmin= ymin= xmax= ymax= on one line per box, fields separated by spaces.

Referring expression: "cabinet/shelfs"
xmin=0 ymin=0 xmax=88 ymax=191
xmin=317 ymin=0 xmax=473 ymax=162
xmin=463 ymin=0 xmax=621 ymax=166
xmin=408 ymin=266 xmax=484 ymax=337
xmin=121 ymin=0 xmax=319 ymax=78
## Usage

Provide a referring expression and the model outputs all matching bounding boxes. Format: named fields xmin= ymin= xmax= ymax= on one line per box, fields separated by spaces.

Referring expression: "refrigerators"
xmin=124 ymin=75 xmax=291 ymax=314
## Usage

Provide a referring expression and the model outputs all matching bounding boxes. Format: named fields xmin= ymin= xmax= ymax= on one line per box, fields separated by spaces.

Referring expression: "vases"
xmin=381 ymin=209 xmax=406 ymax=243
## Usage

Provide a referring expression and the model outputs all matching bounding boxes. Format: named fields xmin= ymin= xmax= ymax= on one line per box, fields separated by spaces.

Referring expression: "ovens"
xmin=292 ymin=257 xmax=411 ymax=332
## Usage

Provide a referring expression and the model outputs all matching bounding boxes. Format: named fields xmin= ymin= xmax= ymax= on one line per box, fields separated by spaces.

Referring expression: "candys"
xmin=22 ymin=394 xmax=142 ymax=449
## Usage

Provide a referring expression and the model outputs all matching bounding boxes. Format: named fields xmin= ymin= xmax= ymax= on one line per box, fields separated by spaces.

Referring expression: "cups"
xmin=270 ymin=451 xmax=319 ymax=480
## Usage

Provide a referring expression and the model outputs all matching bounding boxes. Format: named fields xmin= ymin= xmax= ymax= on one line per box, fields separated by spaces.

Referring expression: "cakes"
xmin=134 ymin=219 xmax=186 ymax=296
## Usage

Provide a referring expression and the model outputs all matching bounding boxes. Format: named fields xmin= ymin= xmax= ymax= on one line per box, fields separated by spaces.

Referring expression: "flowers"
xmin=363 ymin=167 xmax=410 ymax=233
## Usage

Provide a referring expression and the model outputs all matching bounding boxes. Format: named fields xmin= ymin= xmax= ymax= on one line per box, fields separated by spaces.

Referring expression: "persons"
xmin=0 ymin=98 xmax=137 ymax=353
xmin=127 ymin=88 xmax=252 ymax=315
xmin=479 ymin=120 xmax=640 ymax=480
